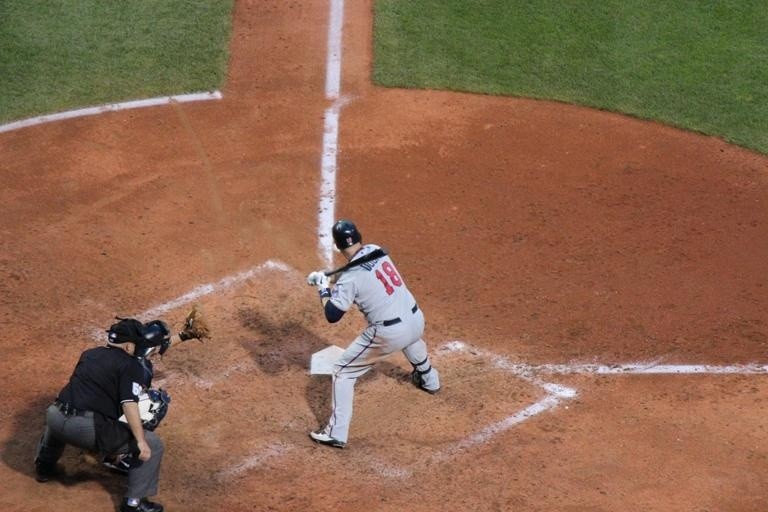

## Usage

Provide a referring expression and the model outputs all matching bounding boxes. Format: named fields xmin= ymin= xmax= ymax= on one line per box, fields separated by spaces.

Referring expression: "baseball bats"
xmin=324 ymin=247 xmax=389 ymax=275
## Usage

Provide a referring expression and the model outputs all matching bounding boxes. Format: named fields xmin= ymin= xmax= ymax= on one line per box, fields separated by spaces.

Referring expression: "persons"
xmin=306 ymin=217 xmax=440 ymax=448
xmin=102 ymin=303 xmax=213 ymax=473
xmin=32 ymin=316 xmax=165 ymax=510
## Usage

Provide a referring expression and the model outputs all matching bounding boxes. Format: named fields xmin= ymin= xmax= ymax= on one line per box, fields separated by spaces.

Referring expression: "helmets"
xmin=143 ymin=320 xmax=170 ymax=355
xmin=333 ymin=219 xmax=360 ymax=250
xmin=108 ymin=318 xmax=145 ymax=355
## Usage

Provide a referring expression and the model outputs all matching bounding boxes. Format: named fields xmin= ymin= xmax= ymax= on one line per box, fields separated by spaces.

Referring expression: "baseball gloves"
xmin=179 ymin=302 xmax=212 ymax=344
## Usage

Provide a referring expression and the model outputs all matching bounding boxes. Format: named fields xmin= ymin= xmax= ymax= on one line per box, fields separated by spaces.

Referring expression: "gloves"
xmin=316 ymin=273 xmax=330 ymax=291
xmin=307 ymin=271 xmax=324 ymax=285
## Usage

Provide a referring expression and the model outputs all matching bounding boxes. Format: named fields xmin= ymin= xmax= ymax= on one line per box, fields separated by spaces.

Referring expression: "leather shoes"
xmin=37 ymin=460 xmax=64 ymax=482
xmin=120 ymin=499 xmax=164 ymax=512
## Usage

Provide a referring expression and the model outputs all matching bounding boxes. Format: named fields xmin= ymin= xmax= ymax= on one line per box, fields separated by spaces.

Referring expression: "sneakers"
xmin=412 ymin=369 xmax=440 ymax=394
xmin=103 ymin=456 xmax=134 ymax=473
xmin=309 ymin=428 xmax=346 ymax=449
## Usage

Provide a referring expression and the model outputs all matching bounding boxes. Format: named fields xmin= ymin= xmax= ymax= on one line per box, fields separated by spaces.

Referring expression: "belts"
xmin=383 ymin=303 xmax=418 ymax=326
xmin=52 ymin=398 xmax=89 ymax=418
